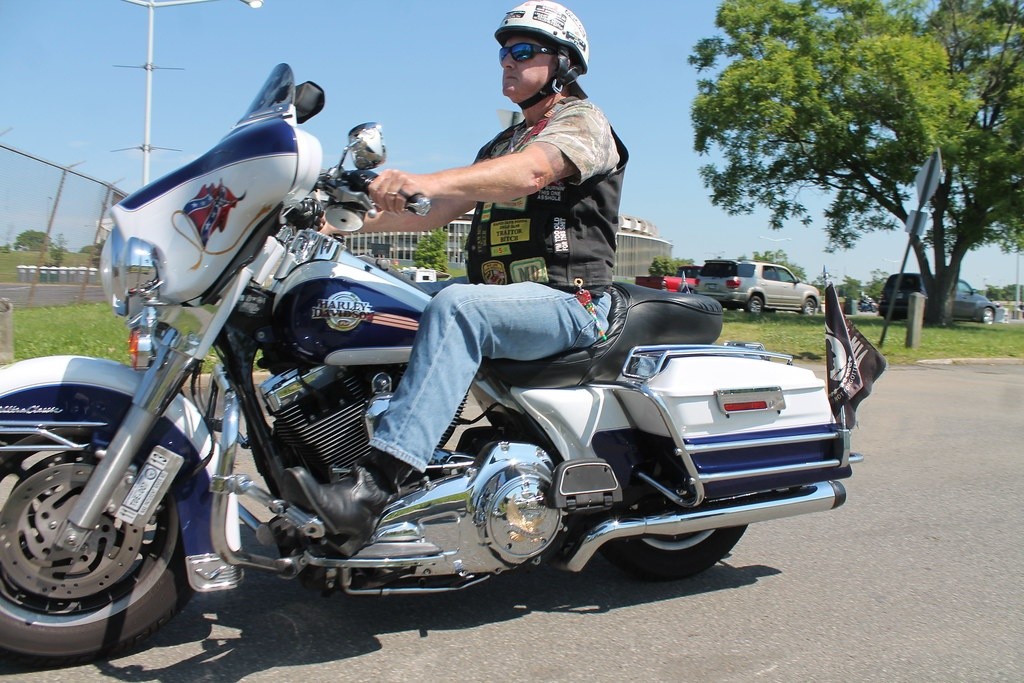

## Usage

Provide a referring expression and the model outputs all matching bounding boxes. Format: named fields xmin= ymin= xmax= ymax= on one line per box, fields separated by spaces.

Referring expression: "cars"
xmin=878 ymin=273 xmax=997 ymax=325
xmin=693 ymin=259 xmax=822 ymax=315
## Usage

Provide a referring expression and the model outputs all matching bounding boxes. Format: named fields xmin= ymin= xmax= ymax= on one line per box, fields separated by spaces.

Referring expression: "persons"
xmin=280 ymin=1 xmax=629 ymax=559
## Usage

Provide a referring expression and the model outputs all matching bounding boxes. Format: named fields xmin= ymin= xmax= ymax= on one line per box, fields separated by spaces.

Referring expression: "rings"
xmin=398 ymin=189 xmax=410 ymax=199
xmin=386 ymin=191 xmax=397 ymax=195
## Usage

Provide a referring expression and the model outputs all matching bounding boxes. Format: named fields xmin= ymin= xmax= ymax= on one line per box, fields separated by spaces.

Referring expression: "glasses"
xmin=499 ymin=43 xmax=557 ymax=62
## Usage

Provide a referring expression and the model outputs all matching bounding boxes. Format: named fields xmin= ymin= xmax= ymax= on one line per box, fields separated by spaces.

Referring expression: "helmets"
xmin=494 ymin=0 xmax=589 ymax=75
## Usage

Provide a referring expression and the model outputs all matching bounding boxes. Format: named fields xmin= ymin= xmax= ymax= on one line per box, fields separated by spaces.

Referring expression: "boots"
xmin=281 ymin=456 xmax=392 ymax=556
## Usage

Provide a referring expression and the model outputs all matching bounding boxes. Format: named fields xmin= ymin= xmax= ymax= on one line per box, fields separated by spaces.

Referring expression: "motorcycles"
xmin=1 ymin=63 xmax=866 ymax=661
xmin=857 ymin=298 xmax=877 ymax=313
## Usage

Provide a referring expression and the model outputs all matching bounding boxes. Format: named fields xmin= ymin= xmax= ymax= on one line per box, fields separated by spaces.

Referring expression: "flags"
xmin=825 ymin=283 xmax=887 ymax=429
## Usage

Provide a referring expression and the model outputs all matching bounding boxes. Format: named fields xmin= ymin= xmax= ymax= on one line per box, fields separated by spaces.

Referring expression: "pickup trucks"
xmin=635 ymin=265 xmax=704 ymax=294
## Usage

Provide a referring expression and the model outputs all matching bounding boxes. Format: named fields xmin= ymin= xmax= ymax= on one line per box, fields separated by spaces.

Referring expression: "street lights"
xmin=883 ymin=259 xmax=899 ymax=273
xmin=758 ymin=234 xmax=793 ymax=262
xmin=143 ymin=0 xmax=262 ymax=186
